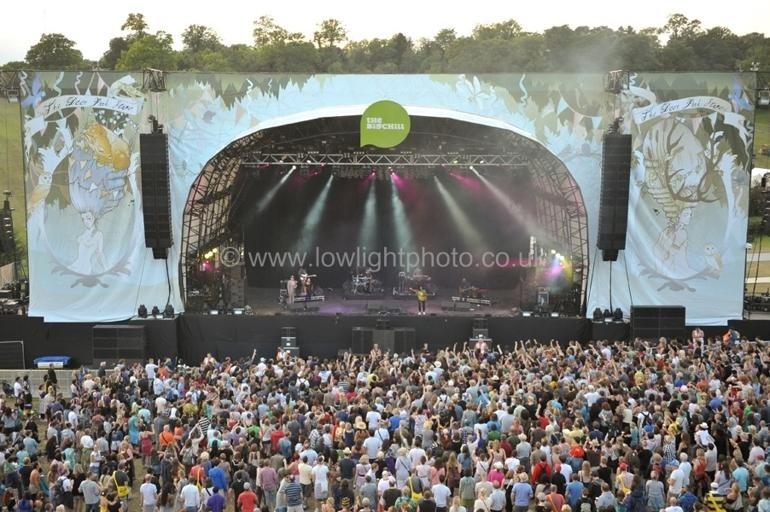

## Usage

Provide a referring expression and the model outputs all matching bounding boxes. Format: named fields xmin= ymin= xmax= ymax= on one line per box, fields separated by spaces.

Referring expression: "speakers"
xmin=441 ymin=302 xmax=471 ymax=311
xmin=630 ymin=305 xmax=685 ymax=343
xmin=351 ymin=327 xmax=373 ymax=355
xmin=468 ymin=337 xmax=493 ymax=350
xmin=728 ymin=320 xmax=769 ymax=341
xmin=277 ymin=346 xmax=301 ymax=359
xmin=367 ymin=302 xmax=401 ymax=313
xmin=289 ymin=301 xmax=319 ymax=313
xmin=0 ymin=340 xmax=25 ymax=369
xmin=92 ymin=324 xmax=146 ymax=368
xmin=140 ymin=134 xmax=174 ymax=258
xmin=596 ymin=134 xmax=632 ymax=262
xmin=394 ymin=327 xmax=417 ymax=354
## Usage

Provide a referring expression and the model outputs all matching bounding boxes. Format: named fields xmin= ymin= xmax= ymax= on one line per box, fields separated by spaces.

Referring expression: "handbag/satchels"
xmin=117 ymin=487 xmax=129 ymax=497
xmin=622 ymin=488 xmax=630 ymax=493
xmin=412 ymin=493 xmax=422 ymax=500
xmin=196 ymin=483 xmax=202 ymax=491
xmin=716 ymin=508 xmax=726 ymax=512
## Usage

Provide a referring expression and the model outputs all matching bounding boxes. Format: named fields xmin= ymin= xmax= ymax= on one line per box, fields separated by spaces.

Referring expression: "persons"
xmin=0 ymin=270 xmax=769 ymax=511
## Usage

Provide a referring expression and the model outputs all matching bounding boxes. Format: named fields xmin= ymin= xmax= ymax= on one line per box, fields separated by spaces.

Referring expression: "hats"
xmin=357 ymin=422 xmax=366 ymax=430
xmin=710 ymin=482 xmax=718 ymax=488
xmin=620 ymin=463 xmax=628 ymax=470
xmin=700 ymin=422 xmax=708 ymax=428
xmin=670 ymin=460 xmax=679 ymax=466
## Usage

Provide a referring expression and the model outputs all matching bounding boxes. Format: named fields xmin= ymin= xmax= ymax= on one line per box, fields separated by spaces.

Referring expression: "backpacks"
xmin=641 ymin=412 xmax=649 ymax=428
xmin=540 ymin=464 xmax=548 ymax=483
